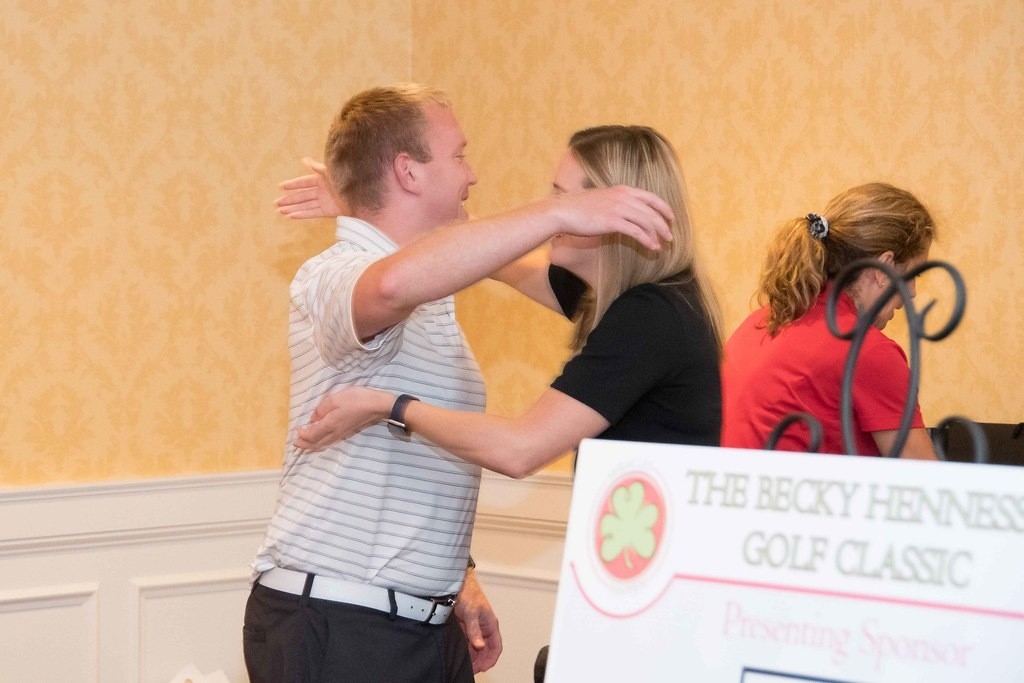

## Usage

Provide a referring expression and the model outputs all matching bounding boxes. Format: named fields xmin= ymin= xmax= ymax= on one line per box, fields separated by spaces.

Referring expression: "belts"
xmin=257 ymin=569 xmax=454 ymax=625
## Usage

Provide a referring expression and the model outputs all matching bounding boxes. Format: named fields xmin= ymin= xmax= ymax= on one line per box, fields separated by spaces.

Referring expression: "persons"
xmin=243 ymin=82 xmax=677 ymax=683
xmin=721 ymin=180 xmax=950 ymax=461
xmin=274 ymin=122 xmax=723 ymax=486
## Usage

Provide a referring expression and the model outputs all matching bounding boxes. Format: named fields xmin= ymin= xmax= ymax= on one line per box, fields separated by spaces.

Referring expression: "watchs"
xmin=386 ymin=392 xmax=421 ymax=442
